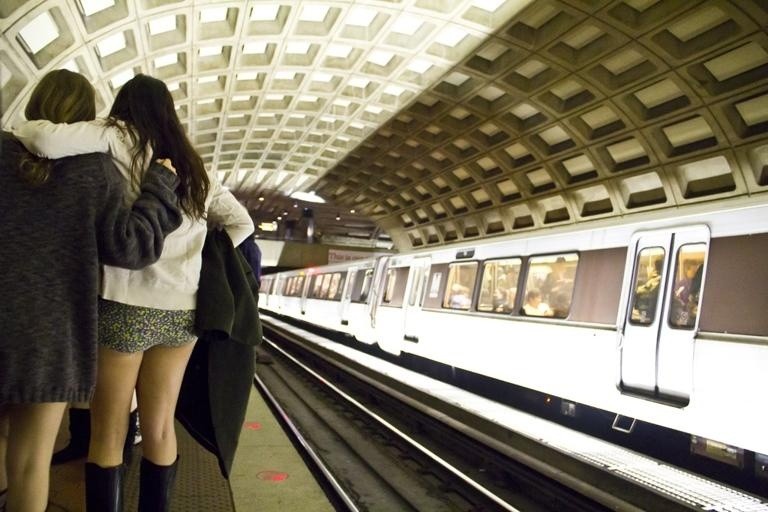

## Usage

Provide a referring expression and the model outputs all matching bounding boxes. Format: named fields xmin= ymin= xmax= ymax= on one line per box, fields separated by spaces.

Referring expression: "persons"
xmin=2 ymin=69 xmax=255 ymax=512
xmin=448 ymin=254 xmax=704 ymax=327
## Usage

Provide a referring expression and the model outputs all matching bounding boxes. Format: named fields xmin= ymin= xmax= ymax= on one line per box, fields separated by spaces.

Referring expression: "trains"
xmin=258 ymin=203 xmax=768 ymax=512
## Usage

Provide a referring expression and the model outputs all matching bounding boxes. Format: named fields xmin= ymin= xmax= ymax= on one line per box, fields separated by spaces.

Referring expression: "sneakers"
xmin=132 ymin=411 xmax=142 ymax=445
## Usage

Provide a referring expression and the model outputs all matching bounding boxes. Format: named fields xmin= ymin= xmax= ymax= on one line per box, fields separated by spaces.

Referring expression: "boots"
xmin=52 ymin=407 xmax=91 ymax=463
xmin=122 ymin=407 xmax=138 ymax=467
